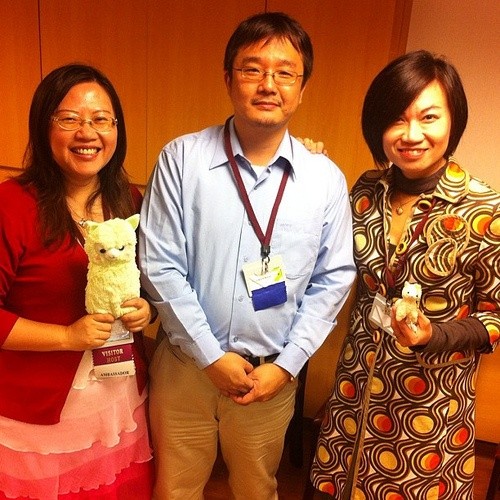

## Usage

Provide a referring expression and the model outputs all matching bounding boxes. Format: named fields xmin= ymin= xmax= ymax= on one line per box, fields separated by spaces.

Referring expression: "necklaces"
xmin=396 ymin=192 xmax=415 ymax=215
xmin=67 ymin=201 xmax=86 ymax=228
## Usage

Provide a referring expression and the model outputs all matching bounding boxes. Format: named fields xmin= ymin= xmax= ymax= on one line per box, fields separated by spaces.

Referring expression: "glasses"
xmin=49 ymin=112 xmax=118 ymax=133
xmin=229 ymin=65 xmax=302 ymax=87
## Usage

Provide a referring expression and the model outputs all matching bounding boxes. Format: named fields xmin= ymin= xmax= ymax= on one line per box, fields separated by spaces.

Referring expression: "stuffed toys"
xmin=81 ymin=214 xmax=141 ymax=319
xmin=394 ymin=281 xmax=422 ymax=325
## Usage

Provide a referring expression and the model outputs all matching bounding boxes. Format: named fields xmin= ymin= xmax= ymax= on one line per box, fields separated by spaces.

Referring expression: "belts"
xmin=244 ymin=354 xmax=278 ymax=367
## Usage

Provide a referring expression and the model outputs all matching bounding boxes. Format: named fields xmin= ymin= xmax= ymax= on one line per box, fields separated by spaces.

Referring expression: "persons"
xmin=138 ymin=12 xmax=357 ymax=500
xmin=0 ymin=65 xmax=159 ymax=500
xmin=297 ymin=50 xmax=500 ymax=500
